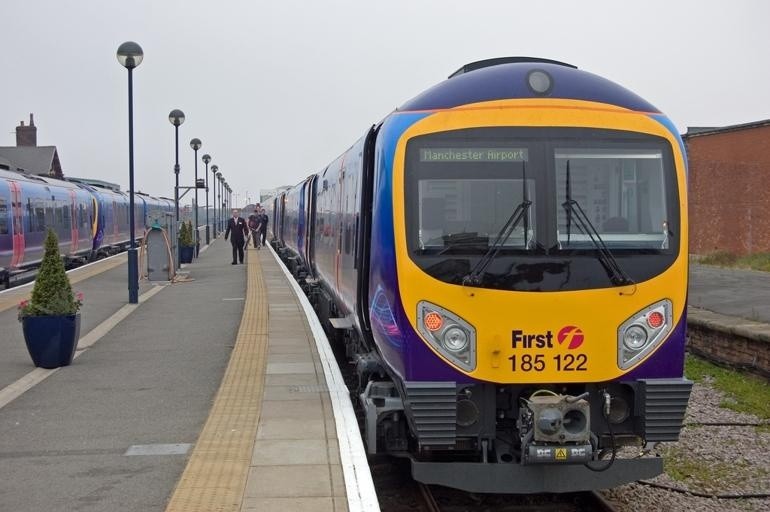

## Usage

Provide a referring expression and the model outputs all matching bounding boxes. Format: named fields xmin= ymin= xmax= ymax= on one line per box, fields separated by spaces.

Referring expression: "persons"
xmin=603 ymin=217 xmax=629 ymax=232
xmin=225 ymin=209 xmax=248 ymax=264
xmin=260 ymin=209 xmax=268 ymax=246
xmin=248 ymin=210 xmax=262 ymax=250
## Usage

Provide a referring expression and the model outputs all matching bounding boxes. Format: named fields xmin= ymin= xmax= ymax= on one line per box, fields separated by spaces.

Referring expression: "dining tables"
xmin=179 ymin=221 xmax=196 ymax=263
xmin=18 ymin=225 xmax=84 ymax=368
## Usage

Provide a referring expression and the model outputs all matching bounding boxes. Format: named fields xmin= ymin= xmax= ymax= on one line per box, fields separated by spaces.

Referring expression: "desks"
xmin=169 ymin=109 xmax=185 ymax=220
xmin=117 ymin=40 xmax=144 ymax=305
xmin=190 ymin=137 xmax=202 ymax=257
xmin=202 ymin=154 xmax=233 ymax=246
